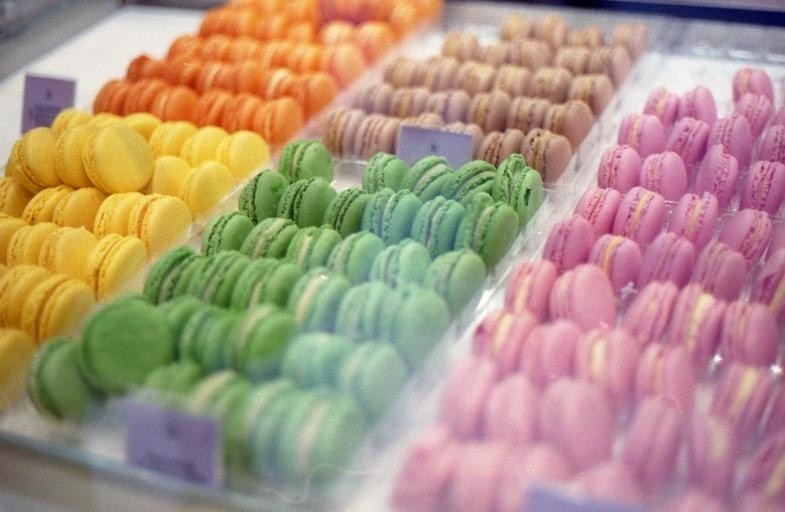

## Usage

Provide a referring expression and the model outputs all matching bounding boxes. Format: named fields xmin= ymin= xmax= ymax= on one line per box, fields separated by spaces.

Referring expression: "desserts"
xmin=0 ymin=1 xmax=785 ymax=512
